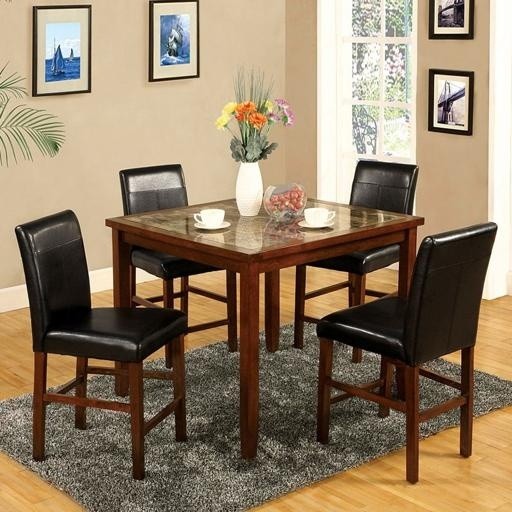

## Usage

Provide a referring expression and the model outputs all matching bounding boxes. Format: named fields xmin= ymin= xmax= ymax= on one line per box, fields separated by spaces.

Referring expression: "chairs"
xmin=289 ymin=154 xmax=423 ymax=361
xmin=13 ymin=208 xmax=195 ymax=483
xmin=117 ymin=161 xmax=240 ymax=370
xmin=316 ymin=216 xmax=495 ymax=484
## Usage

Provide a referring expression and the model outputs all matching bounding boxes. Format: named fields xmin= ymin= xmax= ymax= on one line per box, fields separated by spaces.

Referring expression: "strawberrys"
xmin=267 ymin=188 xmax=303 ymax=211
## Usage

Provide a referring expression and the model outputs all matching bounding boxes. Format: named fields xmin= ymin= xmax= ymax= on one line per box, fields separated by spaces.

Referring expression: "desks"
xmin=99 ymin=189 xmax=426 ymax=461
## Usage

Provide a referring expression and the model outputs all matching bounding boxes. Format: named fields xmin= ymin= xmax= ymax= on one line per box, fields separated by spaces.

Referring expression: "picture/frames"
xmin=30 ymin=4 xmax=92 ymax=98
xmin=427 ymin=0 xmax=475 ymax=42
xmin=148 ymin=0 xmax=200 ymax=83
xmin=426 ymin=67 xmax=476 ymax=137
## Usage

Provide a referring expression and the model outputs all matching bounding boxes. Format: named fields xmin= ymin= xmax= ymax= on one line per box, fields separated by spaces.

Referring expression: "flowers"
xmin=214 ymin=59 xmax=296 ymax=167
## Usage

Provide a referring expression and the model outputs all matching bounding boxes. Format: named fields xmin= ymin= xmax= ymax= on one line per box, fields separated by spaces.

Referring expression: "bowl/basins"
xmin=264 ymin=182 xmax=307 ymax=221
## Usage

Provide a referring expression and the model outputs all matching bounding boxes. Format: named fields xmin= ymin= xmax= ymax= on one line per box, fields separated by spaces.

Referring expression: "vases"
xmin=235 ymin=159 xmax=266 ymax=218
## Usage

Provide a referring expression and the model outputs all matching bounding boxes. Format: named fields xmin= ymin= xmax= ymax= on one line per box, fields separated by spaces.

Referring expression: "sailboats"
xmin=164 ymin=22 xmax=184 ymax=56
xmin=67 ymin=47 xmax=74 ymax=62
xmin=49 ymin=44 xmax=67 ymax=76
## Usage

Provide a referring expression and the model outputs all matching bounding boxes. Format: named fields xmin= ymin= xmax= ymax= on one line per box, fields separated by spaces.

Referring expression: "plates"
xmin=193 ymin=222 xmax=232 ymax=231
xmin=299 ymin=220 xmax=334 ymax=230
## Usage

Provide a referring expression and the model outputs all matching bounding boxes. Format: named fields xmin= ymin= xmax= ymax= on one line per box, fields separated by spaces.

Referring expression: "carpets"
xmin=0 ymin=319 xmax=511 ymax=510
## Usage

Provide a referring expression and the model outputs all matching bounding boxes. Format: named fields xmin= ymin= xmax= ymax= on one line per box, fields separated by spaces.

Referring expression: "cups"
xmin=194 ymin=209 xmax=225 ymax=227
xmin=303 ymin=208 xmax=336 ymax=225
